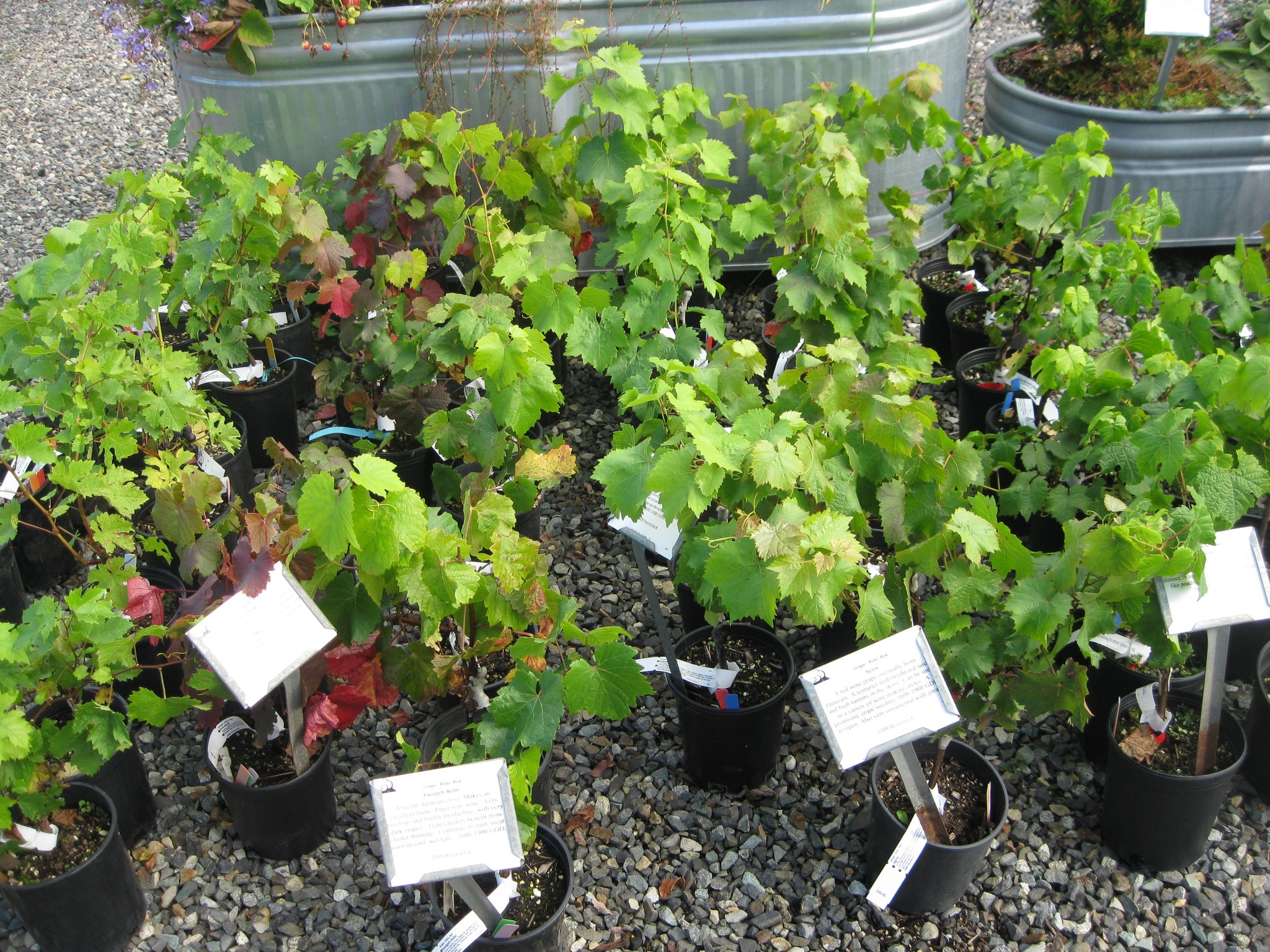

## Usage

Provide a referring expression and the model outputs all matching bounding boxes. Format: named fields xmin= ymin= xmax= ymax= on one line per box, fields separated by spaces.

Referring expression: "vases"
xmin=167 ymin=0 xmax=972 ymax=268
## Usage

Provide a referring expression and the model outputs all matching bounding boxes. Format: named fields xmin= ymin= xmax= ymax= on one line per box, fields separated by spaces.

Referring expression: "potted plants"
xmin=0 ymin=0 xmax=1270 ymax=952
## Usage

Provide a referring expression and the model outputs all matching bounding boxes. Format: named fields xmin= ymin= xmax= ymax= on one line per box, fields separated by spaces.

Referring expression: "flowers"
xmin=101 ymin=0 xmax=375 ymax=97
